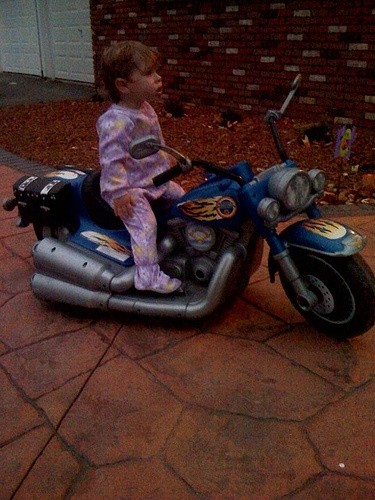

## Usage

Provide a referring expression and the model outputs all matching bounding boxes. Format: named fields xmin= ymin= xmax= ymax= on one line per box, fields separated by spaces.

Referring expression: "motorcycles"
xmin=3 ymin=73 xmax=375 ymax=339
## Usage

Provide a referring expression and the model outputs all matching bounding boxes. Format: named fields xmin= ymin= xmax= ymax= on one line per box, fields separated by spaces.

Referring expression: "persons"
xmin=95 ymin=39 xmax=187 ymax=295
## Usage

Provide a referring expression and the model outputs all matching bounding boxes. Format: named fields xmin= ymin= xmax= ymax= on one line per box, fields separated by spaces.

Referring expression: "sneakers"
xmin=134 ymin=270 xmax=181 ymax=293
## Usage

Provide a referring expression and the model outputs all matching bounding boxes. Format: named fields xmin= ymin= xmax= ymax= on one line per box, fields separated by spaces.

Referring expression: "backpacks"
xmin=13 ymin=166 xmax=86 ymax=226
